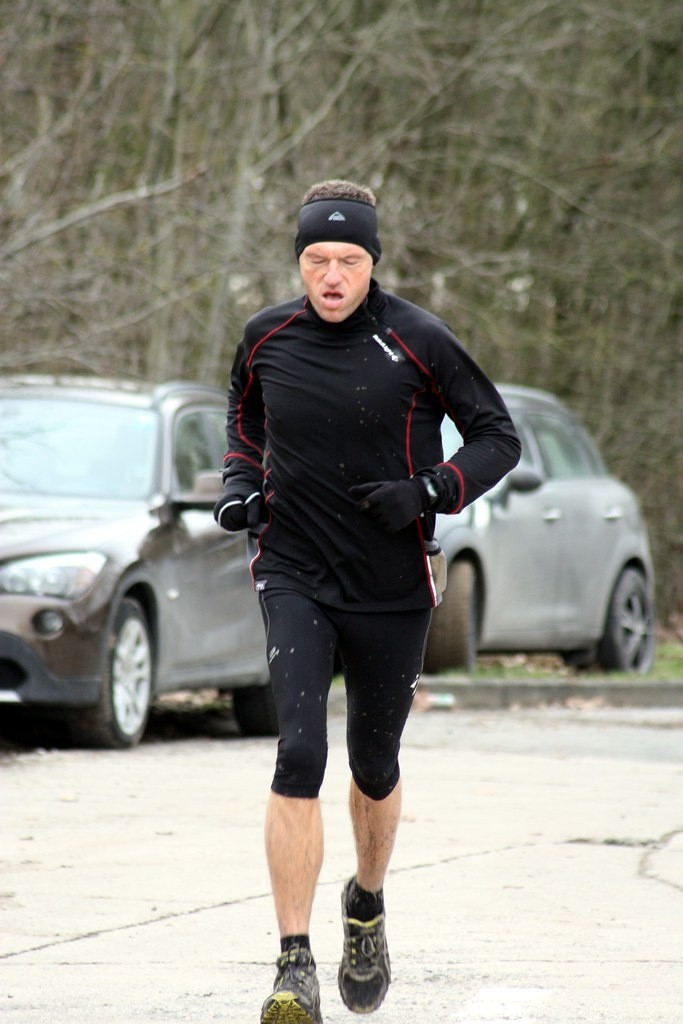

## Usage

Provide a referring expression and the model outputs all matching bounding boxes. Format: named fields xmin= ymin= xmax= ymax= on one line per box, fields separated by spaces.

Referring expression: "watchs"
xmin=422 ymin=475 xmax=438 ymax=504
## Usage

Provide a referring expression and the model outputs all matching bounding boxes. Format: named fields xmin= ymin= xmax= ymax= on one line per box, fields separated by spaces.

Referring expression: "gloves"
xmin=346 ymin=474 xmax=433 ymax=537
xmin=214 ymin=481 xmax=269 ymax=531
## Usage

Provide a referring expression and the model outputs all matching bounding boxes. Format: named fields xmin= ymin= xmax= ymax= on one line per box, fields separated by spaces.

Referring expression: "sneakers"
xmin=260 ymin=943 xmax=323 ymax=1024
xmin=337 ymin=875 xmax=392 ymax=1014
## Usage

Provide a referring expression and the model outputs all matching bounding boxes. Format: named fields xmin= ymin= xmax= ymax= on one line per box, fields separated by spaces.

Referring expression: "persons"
xmin=213 ymin=181 xmax=522 ymax=1024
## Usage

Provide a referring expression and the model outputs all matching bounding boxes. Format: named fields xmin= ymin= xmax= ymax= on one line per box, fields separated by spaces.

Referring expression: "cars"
xmin=424 ymin=383 xmax=656 ymax=677
xmin=1 ymin=376 xmax=278 ymax=748
